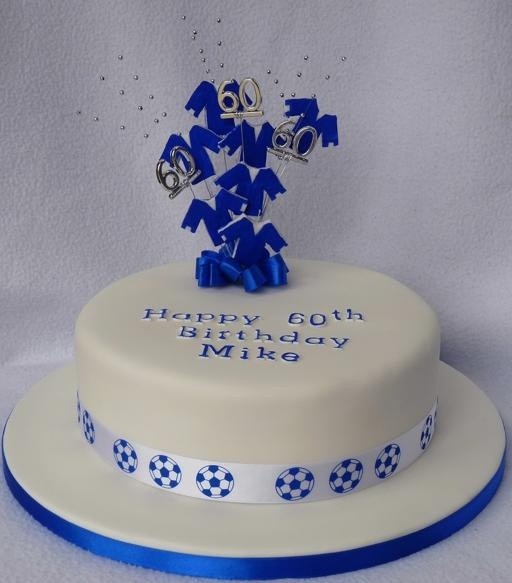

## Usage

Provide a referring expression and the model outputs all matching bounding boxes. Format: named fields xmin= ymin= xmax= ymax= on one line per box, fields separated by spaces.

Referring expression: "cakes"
xmin=1 ymin=78 xmax=507 ymax=581
